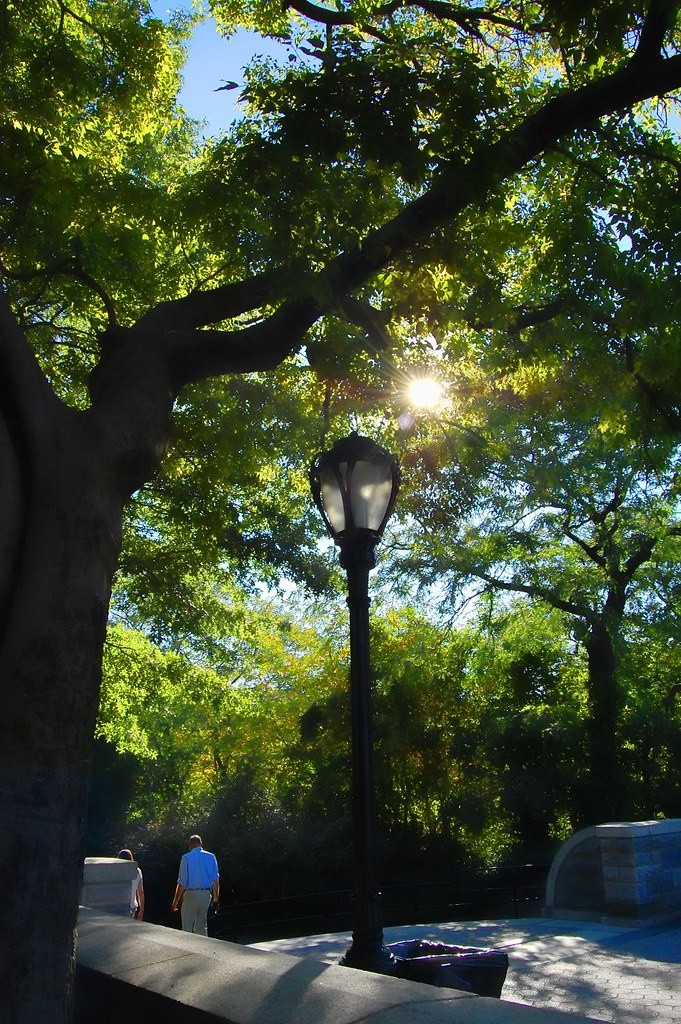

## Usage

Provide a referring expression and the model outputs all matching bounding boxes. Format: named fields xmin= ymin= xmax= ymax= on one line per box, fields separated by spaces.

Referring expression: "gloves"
xmin=211 ymin=902 xmax=219 ymax=914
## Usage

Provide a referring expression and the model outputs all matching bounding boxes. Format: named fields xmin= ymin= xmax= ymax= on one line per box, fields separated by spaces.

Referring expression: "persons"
xmin=118 ymin=849 xmax=145 ymax=921
xmin=172 ymin=835 xmax=221 ymax=937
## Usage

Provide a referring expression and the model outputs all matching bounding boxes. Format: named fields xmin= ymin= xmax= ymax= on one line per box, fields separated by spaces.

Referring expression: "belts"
xmin=185 ymin=886 xmax=210 ymax=890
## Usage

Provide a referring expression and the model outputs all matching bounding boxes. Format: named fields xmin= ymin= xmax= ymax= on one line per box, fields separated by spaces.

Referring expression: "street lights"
xmin=308 ymin=428 xmax=399 ymax=976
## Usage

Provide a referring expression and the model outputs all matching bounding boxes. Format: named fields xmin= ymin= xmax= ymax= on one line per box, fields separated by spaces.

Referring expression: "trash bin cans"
xmin=380 ymin=938 xmax=508 ymax=1000
